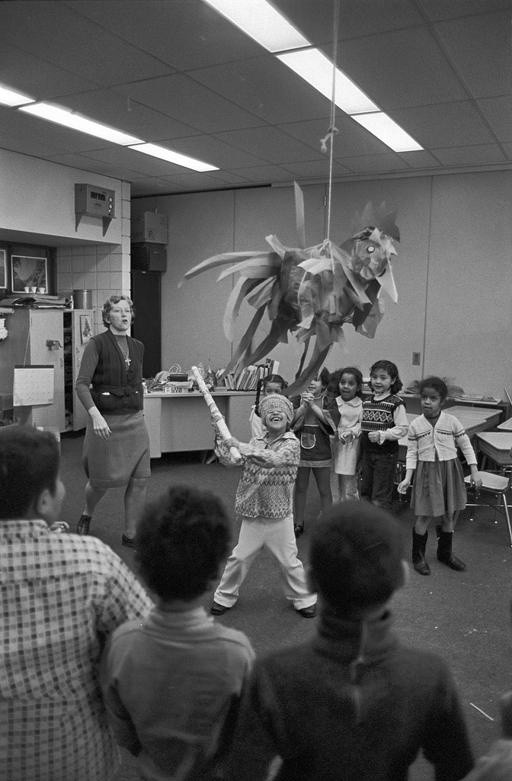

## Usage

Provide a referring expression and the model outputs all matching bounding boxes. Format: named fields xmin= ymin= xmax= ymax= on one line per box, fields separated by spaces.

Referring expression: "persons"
xmin=0 ymin=423 xmax=155 ymax=781
xmin=249 ymin=373 xmax=287 ymax=438
xmin=287 ymin=365 xmax=341 ymax=537
xmin=339 ymin=359 xmax=410 ymax=514
xmin=328 ymin=366 xmax=363 ymax=502
xmin=201 ymin=499 xmax=475 ymax=781
xmin=405 ymin=394 xmax=482 ymax=575
xmin=99 ymin=484 xmax=255 ymax=781
xmin=209 ymin=394 xmax=316 ymax=618
xmin=76 ymin=294 xmax=151 ymax=549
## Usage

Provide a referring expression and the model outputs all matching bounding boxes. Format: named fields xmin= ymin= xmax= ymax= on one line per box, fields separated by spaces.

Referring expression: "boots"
xmin=411 ymin=526 xmax=430 ymax=576
xmin=434 ymin=524 xmax=467 ymax=571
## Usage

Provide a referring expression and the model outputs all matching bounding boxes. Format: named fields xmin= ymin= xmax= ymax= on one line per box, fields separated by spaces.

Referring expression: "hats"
xmin=259 ymin=394 xmax=295 ymax=425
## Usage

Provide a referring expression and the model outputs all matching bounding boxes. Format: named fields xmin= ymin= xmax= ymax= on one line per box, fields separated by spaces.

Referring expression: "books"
xmin=224 ymin=357 xmax=279 ymax=391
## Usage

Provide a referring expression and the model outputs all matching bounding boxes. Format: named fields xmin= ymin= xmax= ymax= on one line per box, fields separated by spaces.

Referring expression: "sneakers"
xmin=294 ymin=521 xmax=305 ymax=539
xmin=301 ymin=604 xmax=317 ymax=617
xmin=209 ymin=599 xmax=224 ymax=615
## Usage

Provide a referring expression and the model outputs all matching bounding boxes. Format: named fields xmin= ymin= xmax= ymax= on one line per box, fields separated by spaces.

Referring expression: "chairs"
xmin=453 ymin=447 xmax=512 ymax=549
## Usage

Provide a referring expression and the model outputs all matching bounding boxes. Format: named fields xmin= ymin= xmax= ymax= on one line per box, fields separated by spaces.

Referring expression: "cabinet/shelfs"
xmin=29 ymin=309 xmax=65 ymax=433
xmin=71 ymin=311 xmax=95 ymax=431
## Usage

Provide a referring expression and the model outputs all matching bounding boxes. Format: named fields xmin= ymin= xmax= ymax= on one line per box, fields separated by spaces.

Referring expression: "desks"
xmin=394 ymin=413 xmax=487 ymax=503
xmin=477 ymin=432 xmax=512 ymax=515
xmin=143 ymin=383 xmax=264 ymax=464
xmin=444 ymin=405 xmax=503 ymax=431
xmin=497 ymin=416 xmax=512 ymax=431
